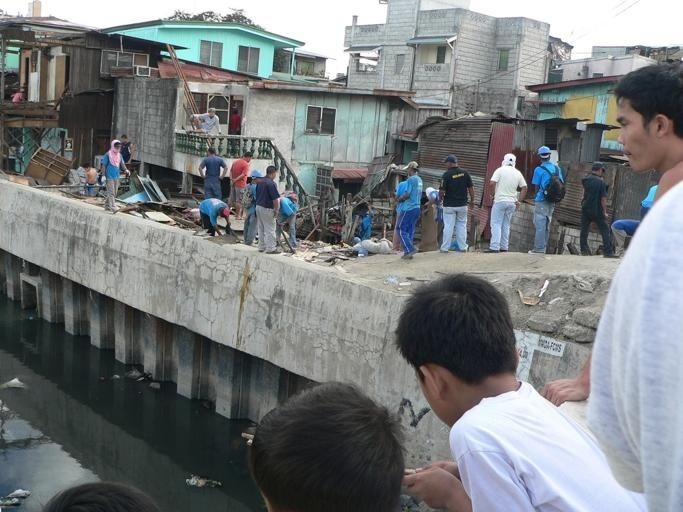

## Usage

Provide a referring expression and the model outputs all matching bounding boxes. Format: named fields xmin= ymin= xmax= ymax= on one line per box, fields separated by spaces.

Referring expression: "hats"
xmin=503 ymin=153 xmax=517 ymax=162
xmin=592 ymin=161 xmax=605 ymax=173
xmin=442 ymin=155 xmax=458 ymax=164
xmin=403 ymin=161 xmax=419 ymax=170
xmin=223 ymin=207 xmax=231 ymax=219
xmin=251 ymin=170 xmax=262 ymax=178
xmin=114 ymin=143 xmax=122 ymax=147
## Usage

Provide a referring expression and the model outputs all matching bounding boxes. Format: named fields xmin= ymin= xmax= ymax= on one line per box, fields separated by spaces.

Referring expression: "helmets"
xmin=537 ymin=146 xmax=552 ymax=156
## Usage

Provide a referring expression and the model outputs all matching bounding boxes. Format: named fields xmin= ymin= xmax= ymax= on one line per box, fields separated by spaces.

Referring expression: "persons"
xmin=483 ymin=153 xmax=528 ymax=255
xmin=198 ymin=147 xmax=228 ymax=199
xmin=276 ymin=194 xmax=297 ymax=248
xmin=43 ymin=480 xmax=168 ymax=511
xmin=199 ymin=198 xmax=232 ymax=237
xmin=587 ymin=178 xmax=683 ymax=511
xmin=230 ymin=151 xmax=253 ymax=220
xmin=255 ymin=166 xmax=282 ymax=254
xmin=231 ymin=109 xmax=240 ymax=134
xmin=441 ymin=154 xmax=475 ymax=253
xmin=528 ymin=145 xmax=565 ymax=255
xmin=581 ymin=163 xmax=620 ymax=258
xmin=101 ymin=140 xmax=130 ymax=213
xmin=538 ymin=59 xmax=683 ymax=406
xmin=431 ymin=185 xmax=445 ymax=248
xmin=396 ymin=273 xmax=647 ymax=511
xmin=120 ymin=134 xmax=134 ymax=163
xmin=394 ymin=160 xmax=423 ymax=259
xmin=250 ymin=379 xmax=406 ymax=511
xmin=11 ymin=88 xmax=26 ymax=103
xmin=82 ymin=163 xmax=98 ymax=196
xmin=241 ymin=169 xmax=261 ymax=245
xmin=190 ymin=108 xmax=221 ymax=134
xmin=393 ymin=182 xmax=407 ymax=251
xmin=361 ymin=206 xmax=375 ymax=240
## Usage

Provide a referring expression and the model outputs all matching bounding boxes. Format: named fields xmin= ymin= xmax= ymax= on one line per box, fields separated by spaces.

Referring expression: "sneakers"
xmin=401 ymin=249 xmax=418 ymax=260
xmin=266 ymin=249 xmax=282 ymax=253
xmin=498 ymin=250 xmax=509 ymax=252
xmin=484 ymin=250 xmax=499 ymax=253
xmin=604 ymin=254 xmax=620 ymax=258
xmin=528 ymin=250 xmax=545 ymax=255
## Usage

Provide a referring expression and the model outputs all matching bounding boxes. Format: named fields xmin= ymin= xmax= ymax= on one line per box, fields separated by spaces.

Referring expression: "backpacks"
xmin=539 ymin=165 xmax=565 ymax=202
xmin=282 ymin=191 xmax=299 ymax=204
